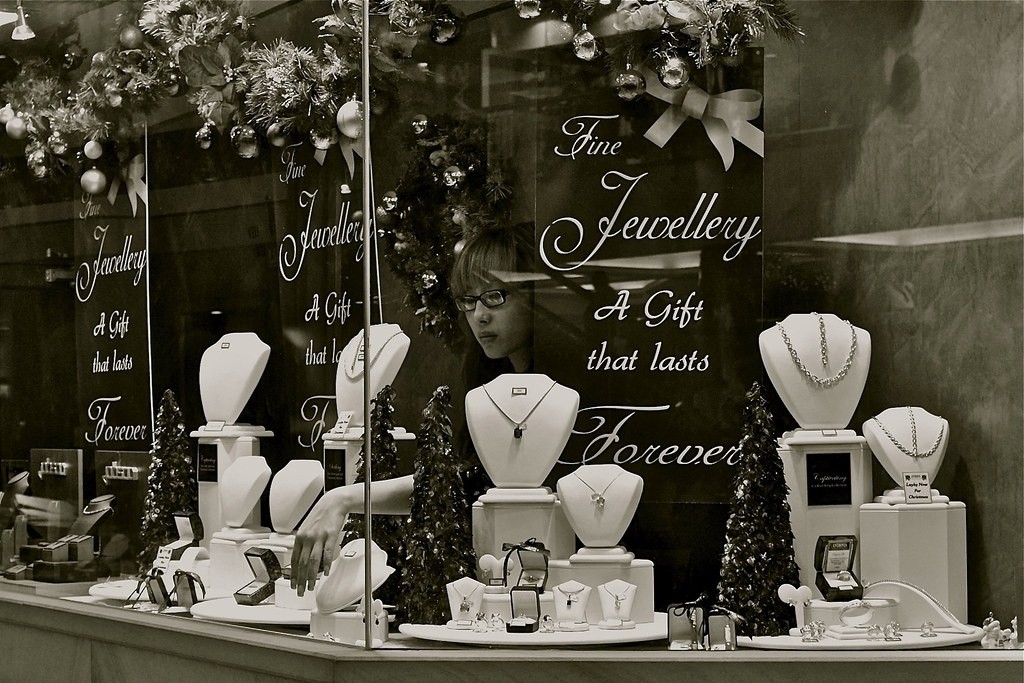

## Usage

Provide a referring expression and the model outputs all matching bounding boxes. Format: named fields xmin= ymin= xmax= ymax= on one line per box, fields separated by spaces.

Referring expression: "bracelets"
xmin=839 ymin=602 xmax=875 ymax=626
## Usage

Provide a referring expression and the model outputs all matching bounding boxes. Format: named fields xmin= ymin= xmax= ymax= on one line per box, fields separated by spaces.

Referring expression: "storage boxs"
xmin=233 ymin=546 xmax=282 ymax=606
xmin=119 ymin=567 xmax=205 ymax=618
xmin=814 ymin=535 xmax=864 ymax=603
xmin=506 ymin=587 xmax=541 ymax=633
xmin=516 ymin=549 xmax=548 ymax=594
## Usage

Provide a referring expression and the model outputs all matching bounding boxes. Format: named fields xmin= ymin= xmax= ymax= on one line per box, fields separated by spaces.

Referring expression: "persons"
xmin=290 ymin=233 xmax=686 ymax=603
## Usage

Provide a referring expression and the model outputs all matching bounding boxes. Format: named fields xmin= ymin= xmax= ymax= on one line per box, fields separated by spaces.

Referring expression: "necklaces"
xmin=775 ymin=313 xmax=857 ymax=387
xmin=558 ymin=586 xmax=585 ymax=605
xmin=872 ymin=406 xmax=944 ymax=458
xmin=343 ymin=330 xmax=404 ymax=379
xmin=453 ymin=584 xmax=479 ymax=612
xmin=83 ymin=505 xmax=115 ymax=518
xmin=604 ymin=583 xmax=631 ymax=607
xmin=574 ymin=471 xmax=625 ymax=508
xmin=483 ymin=381 xmax=557 ymax=439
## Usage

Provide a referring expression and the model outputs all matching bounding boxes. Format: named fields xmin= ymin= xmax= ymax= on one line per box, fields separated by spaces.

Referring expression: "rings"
xmin=518 ymin=613 xmax=527 ymax=619
xmin=837 ymin=570 xmax=851 ymax=580
xmin=922 ymin=622 xmax=936 ymax=637
xmin=529 ymin=575 xmax=539 ymax=581
xmin=868 ymin=621 xmax=900 ymax=639
xmin=801 ymin=621 xmax=826 ymax=639
xmin=474 ymin=612 xmax=502 ymax=620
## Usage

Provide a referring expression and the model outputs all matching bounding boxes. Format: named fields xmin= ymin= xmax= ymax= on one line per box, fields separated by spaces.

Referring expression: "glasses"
xmin=453 ymin=284 xmax=526 ymax=312
